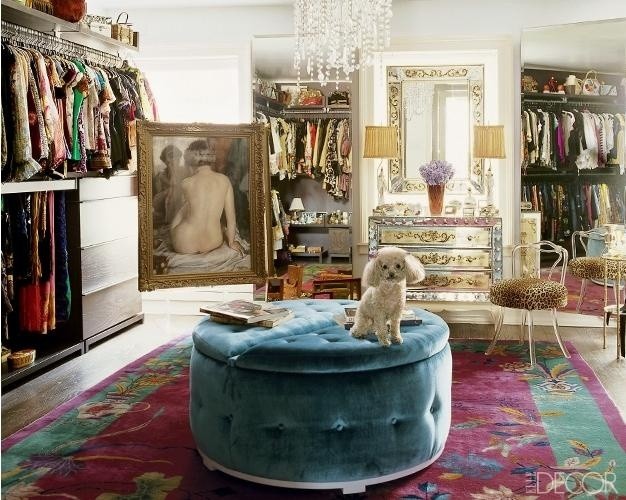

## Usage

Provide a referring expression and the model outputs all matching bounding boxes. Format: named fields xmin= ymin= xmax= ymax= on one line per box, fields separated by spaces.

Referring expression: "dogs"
xmin=349 ymin=246 xmax=426 ymax=348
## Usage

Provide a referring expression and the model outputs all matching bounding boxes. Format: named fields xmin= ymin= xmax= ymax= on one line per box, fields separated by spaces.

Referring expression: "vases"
xmin=428 ymin=183 xmax=447 ymax=215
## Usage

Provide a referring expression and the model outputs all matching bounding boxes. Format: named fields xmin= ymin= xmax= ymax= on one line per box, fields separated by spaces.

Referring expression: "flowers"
xmin=417 ymin=161 xmax=455 ymax=185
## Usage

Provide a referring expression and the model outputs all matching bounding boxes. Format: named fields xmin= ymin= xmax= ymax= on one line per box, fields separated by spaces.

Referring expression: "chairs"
xmin=570 ymin=224 xmax=626 ymax=326
xmin=483 ymin=238 xmax=571 ymax=368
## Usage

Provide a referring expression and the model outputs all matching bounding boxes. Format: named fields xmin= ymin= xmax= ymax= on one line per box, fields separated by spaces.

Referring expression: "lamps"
xmin=404 ymin=81 xmax=433 ymax=123
xmin=292 ymin=0 xmax=393 ymax=95
xmin=361 ymin=125 xmax=402 ymax=209
xmin=288 ymin=197 xmax=303 ymax=219
xmin=473 ymin=125 xmax=508 ymax=212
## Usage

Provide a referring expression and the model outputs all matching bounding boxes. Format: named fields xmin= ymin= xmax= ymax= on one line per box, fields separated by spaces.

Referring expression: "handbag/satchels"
xmin=329 ymin=91 xmax=349 ymax=104
xmin=577 ymin=70 xmax=600 ymax=95
xmin=295 ymin=88 xmax=322 ymax=105
xmin=111 ymin=12 xmax=133 ymax=45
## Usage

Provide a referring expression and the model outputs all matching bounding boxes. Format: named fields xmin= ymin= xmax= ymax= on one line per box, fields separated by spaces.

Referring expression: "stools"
xmin=586 ymin=228 xmax=626 ymax=288
xmin=188 ymin=298 xmax=454 ymax=496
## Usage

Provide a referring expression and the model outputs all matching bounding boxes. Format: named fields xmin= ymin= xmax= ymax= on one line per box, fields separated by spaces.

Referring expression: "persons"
xmin=152 ymin=137 xmax=246 ymax=255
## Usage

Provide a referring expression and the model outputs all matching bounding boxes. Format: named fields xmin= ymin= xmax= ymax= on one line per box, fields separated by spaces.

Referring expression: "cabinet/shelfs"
xmin=366 ymin=215 xmax=504 ymax=306
xmin=62 ymin=174 xmax=143 ymax=340
xmin=521 ymin=212 xmax=543 ymax=279
xmin=597 ymin=253 xmax=626 ymax=363
xmin=284 ymin=212 xmax=351 ymax=262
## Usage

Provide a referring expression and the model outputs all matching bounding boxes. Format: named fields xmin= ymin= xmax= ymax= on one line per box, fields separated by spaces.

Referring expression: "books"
xmin=332 ymin=308 xmax=422 ymax=330
xmin=200 ymin=300 xmax=294 ymax=328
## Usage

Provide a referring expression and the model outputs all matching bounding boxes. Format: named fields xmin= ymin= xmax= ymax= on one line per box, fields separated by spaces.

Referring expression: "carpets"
xmin=541 ymin=268 xmax=625 ymax=319
xmin=0 ymin=334 xmax=626 ymax=500
xmin=257 ymin=263 xmax=353 ymax=299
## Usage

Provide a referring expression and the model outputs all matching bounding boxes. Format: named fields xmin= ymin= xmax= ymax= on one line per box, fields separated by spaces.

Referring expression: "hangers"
xmin=522 ymin=99 xmax=614 ymax=116
xmin=1 ymin=18 xmax=129 ymax=75
xmin=523 ymin=172 xmax=615 ymax=188
xmin=255 ymin=102 xmax=349 ymax=127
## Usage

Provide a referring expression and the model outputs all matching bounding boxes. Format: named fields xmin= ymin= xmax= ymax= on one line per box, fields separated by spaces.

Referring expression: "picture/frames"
xmin=135 ymin=119 xmax=272 ymax=291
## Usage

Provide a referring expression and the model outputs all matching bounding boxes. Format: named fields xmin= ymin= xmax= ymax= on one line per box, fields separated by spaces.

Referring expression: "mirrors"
xmin=520 ymin=38 xmax=625 ymax=327
xmin=238 ymin=36 xmax=367 ymax=302
xmin=386 ymin=63 xmax=485 ymax=194
